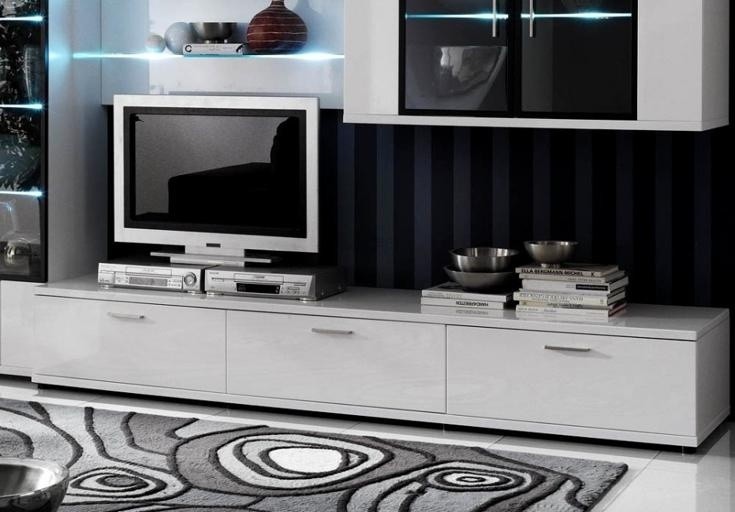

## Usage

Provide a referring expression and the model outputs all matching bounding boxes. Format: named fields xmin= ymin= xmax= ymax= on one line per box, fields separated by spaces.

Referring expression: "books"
xmin=511 ymin=261 xmax=632 ymax=323
xmin=419 ymin=280 xmax=514 ymax=311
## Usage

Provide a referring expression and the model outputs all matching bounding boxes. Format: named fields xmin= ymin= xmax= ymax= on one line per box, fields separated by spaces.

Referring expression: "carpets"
xmin=0 ymin=392 xmax=632 ymax=512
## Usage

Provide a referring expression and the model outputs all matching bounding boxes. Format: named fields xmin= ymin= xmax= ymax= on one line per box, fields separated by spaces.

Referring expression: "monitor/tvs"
xmin=113 ymin=94 xmax=320 ymax=267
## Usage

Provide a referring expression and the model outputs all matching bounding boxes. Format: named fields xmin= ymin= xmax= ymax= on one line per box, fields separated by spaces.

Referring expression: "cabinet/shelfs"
xmin=227 ymin=283 xmax=446 ymax=424
xmin=29 ymin=264 xmax=227 ymax=403
xmin=447 ymin=301 xmax=729 ymax=447
xmin=333 ymin=1 xmax=731 ymax=137
xmin=0 ymin=0 xmax=103 ymax=379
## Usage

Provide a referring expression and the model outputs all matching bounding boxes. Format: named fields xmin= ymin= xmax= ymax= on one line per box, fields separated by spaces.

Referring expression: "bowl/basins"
xmin=451 ymin=248 xmax=518 ymax=272
xmin=187 ymin=21 xmax=237 ymax=45
xmin=524 ymin=239 xmax=578 ymax=267
xmin=443 ymin=263 xmax=518 ymax=292
